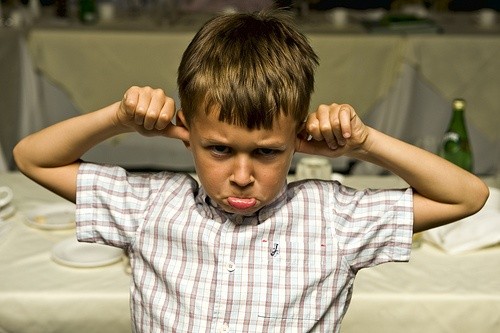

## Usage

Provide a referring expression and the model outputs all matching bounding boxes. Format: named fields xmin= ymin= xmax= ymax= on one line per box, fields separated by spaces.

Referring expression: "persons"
xmin=12 ymin=12 xmax=490 ymax=333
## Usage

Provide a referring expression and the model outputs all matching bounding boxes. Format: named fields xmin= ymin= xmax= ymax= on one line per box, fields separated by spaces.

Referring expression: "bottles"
xmin=441 ymin=99 xmax=472 ymax=173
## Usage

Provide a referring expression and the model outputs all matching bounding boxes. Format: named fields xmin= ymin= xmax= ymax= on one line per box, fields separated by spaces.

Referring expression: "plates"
xmin=27 ymin=203 xmax=75 ymax=229
xmin=52 ymin=237 xmax=123 ymax=267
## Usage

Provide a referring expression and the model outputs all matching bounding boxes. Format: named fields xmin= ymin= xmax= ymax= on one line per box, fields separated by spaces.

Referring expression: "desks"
xmin=23 ymin=21 xmax=500 ymax=172
xmin=0 ymin=171 xmax=499 ymax=333
